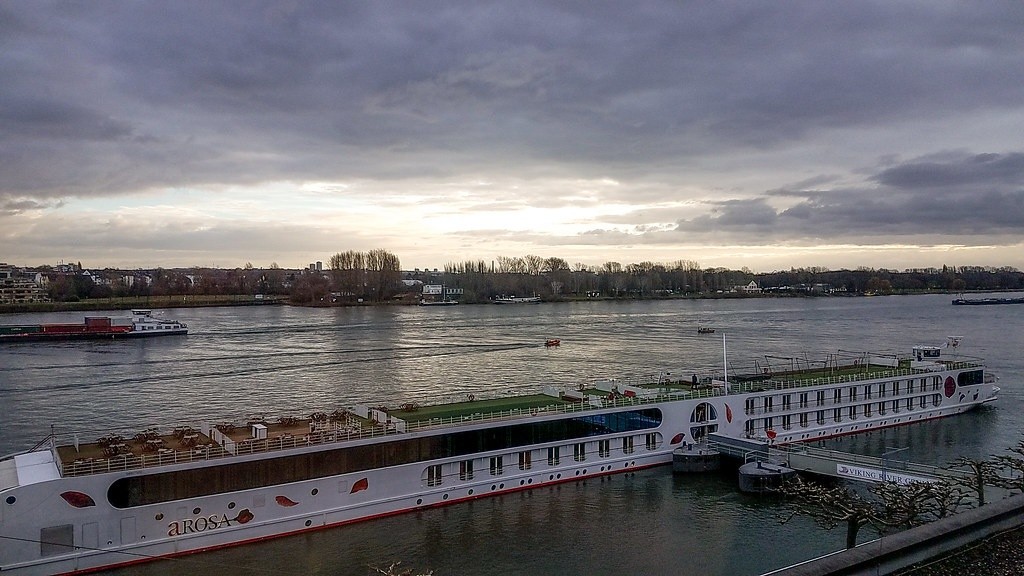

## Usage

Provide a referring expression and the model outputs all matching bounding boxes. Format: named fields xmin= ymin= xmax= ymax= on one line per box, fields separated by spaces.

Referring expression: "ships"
xmin=1 ymin=333 xmax=1019 ymax=575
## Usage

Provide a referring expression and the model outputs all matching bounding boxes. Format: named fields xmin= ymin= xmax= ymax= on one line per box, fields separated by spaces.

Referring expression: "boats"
xmin=950 ymin=297 xmax=1024 ymax=305
xmin=0 ymin=307 xmax=189 ymax=348
xmin=544 ymin=338 xmax=561 ymax=348
xmin=697 ymin=326 xmax=715 ymax=334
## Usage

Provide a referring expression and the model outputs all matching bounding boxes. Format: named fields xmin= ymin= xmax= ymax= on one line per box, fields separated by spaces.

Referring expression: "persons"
xmin=691 ymin=374 xmax=698 ymax=391
